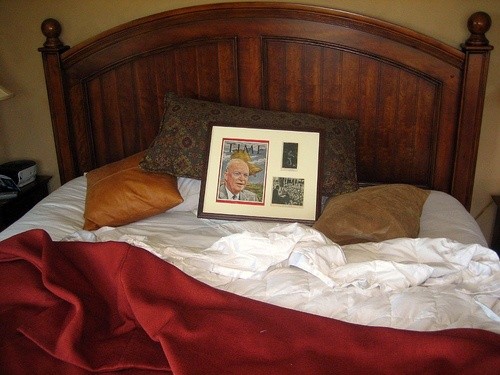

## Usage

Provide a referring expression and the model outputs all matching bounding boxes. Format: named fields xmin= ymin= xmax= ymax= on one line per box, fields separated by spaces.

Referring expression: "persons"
xmin=219 ymin=159 xmax=289 ymax=204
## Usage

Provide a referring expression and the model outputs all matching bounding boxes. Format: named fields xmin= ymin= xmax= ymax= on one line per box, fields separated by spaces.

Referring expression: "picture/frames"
xmin=197 ymin=120 xmax=326 ymax=224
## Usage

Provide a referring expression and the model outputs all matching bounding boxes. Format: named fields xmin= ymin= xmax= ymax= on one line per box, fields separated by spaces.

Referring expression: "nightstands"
xmin=0 ymin=174 xmax=53 ymax=233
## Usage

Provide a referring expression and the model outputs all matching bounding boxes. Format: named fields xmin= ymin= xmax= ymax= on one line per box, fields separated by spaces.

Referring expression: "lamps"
xmin=0 ymin=83 xmax=16 ymax=103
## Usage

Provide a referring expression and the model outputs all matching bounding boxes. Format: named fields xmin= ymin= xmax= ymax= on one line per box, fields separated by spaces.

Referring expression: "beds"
xmin=0 ymin=1 xmax=500 ymax=375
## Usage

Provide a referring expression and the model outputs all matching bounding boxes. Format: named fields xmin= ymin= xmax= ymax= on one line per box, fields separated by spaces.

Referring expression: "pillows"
xmin=138 ymin=92 xmax=360 ymax=197
xmin=315 ymin=184 xmax=431 ymax=246
xmin=84 ymin=149 xmax=184 ymax=231
